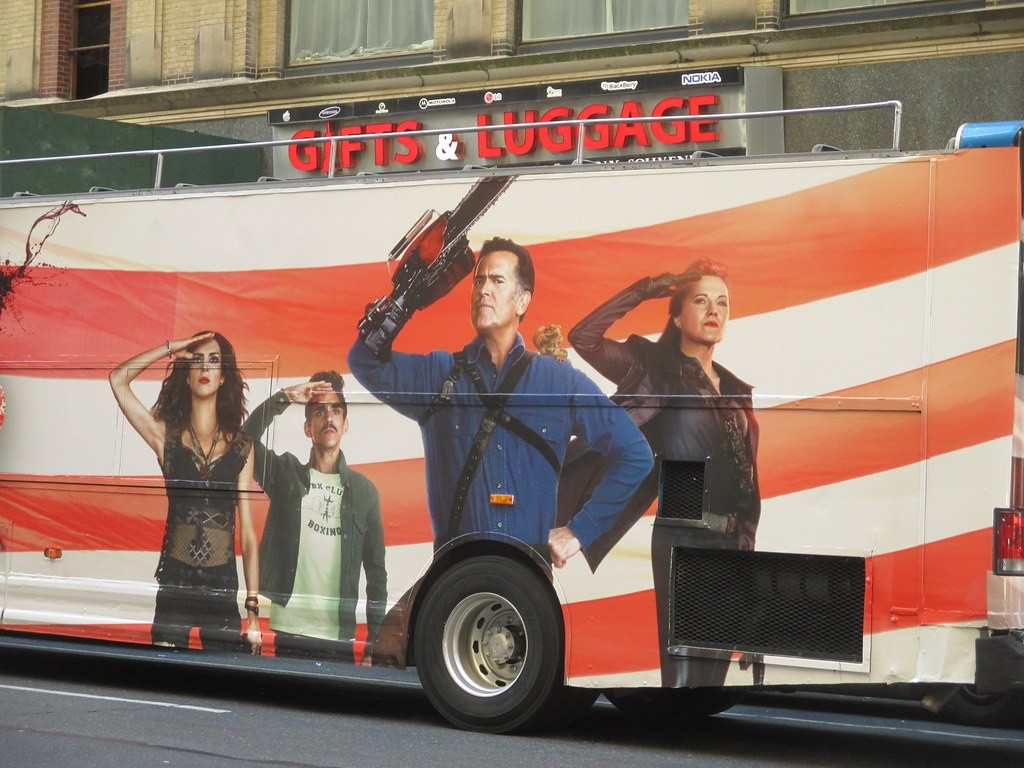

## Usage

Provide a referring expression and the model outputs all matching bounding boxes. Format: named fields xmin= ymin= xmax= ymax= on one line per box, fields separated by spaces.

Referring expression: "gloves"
xmin=632 ymin=271 xmax=704 ymax=299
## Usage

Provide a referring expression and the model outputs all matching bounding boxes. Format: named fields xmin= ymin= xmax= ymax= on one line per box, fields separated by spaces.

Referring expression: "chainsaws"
xmin=356 ymin=175 xmax=520 ymax=363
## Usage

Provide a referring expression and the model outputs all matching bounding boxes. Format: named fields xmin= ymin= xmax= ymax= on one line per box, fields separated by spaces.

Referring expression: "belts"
xmin=698 ymin=514 xmax=742 ymax=533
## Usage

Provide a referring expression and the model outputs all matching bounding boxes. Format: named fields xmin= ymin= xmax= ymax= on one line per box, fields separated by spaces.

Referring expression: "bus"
xmin=0 ymin=90 xmax=1024 ymax=733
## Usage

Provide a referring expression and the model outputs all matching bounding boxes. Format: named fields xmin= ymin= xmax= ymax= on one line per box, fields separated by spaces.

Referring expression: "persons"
xmin=108 ymin=329 xmax=265 ymax=655
xmin=241 ymin=370 xmax=389 ymax=667
xmin=347 ymin=235 xmax=656 ymax=586
xmin=556 ymin=258 xmax=762 ymax=687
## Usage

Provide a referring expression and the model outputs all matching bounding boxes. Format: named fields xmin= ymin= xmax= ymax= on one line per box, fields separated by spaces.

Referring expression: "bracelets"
xmin=164 ymin=339 xmax=172 ymax=358
xmin=244 ymin=597 xmax=260 ymax=616
